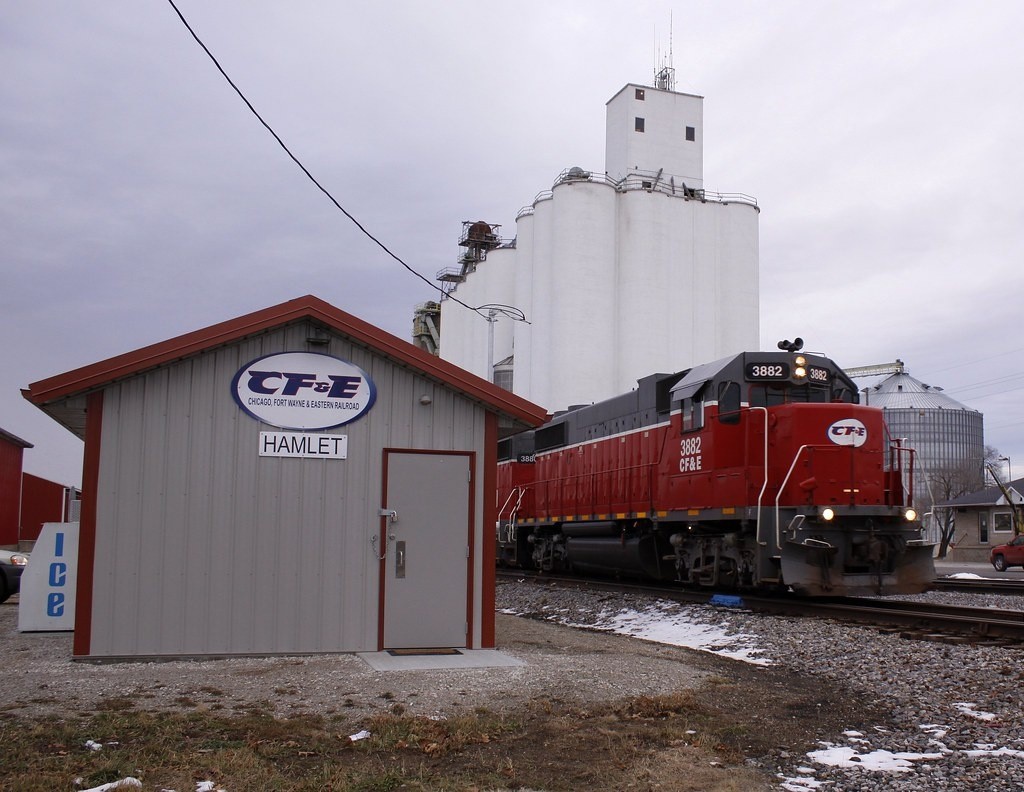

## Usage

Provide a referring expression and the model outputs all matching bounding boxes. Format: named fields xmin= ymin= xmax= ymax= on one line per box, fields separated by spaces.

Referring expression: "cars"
xmin=0 ymin=549 xmax=33 ymax=603
xmin=989 ymin=534 xmax=1024 ymax=573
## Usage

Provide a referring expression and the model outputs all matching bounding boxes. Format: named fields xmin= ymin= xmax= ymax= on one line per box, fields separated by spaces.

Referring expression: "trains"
xmin=494 ymin=337 xmax=943 ymax=605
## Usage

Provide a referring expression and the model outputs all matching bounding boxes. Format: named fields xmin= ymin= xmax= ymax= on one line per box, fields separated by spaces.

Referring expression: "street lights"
xmin=998 ymin=455 xmax=1017 ymax=533
